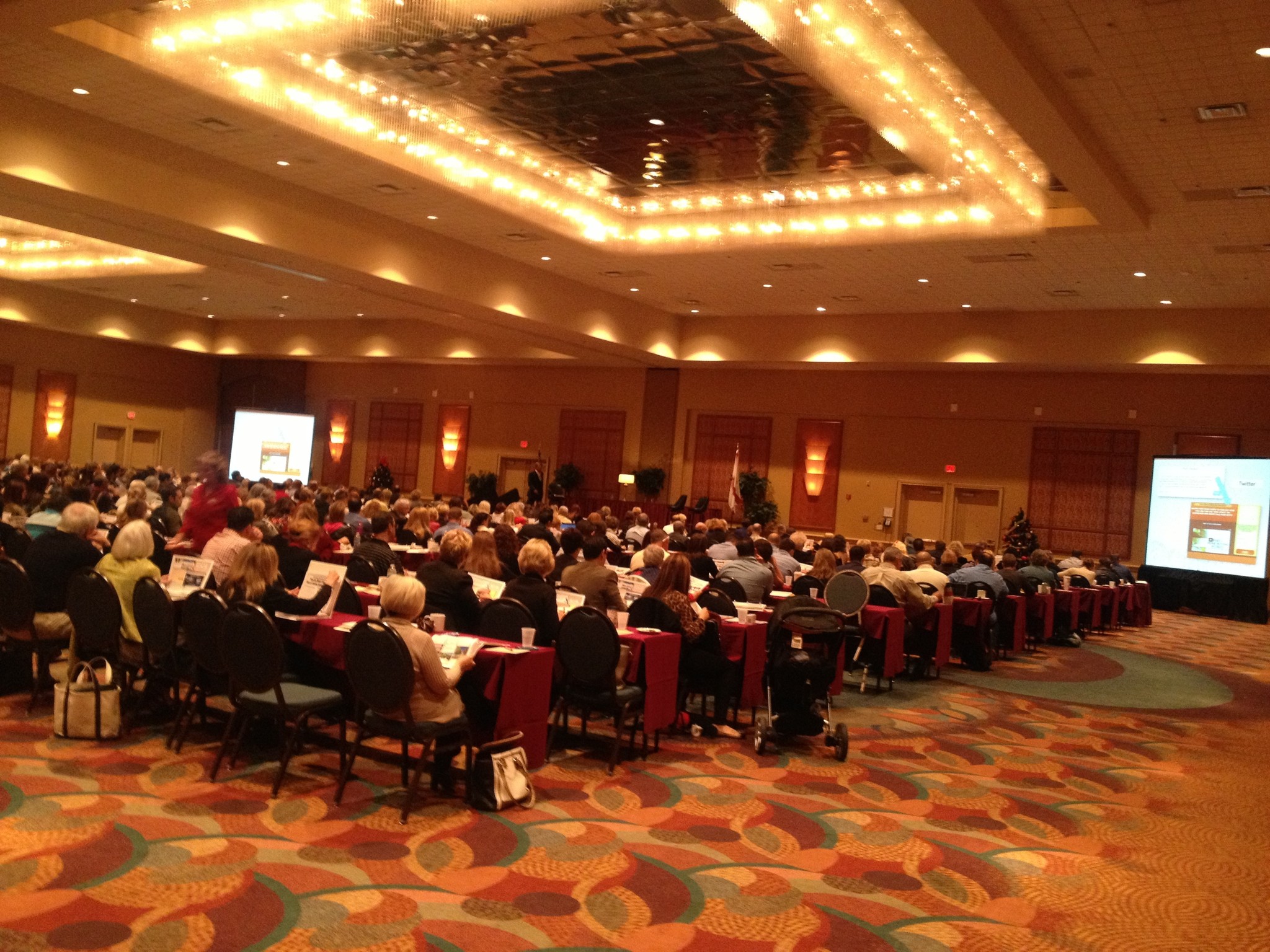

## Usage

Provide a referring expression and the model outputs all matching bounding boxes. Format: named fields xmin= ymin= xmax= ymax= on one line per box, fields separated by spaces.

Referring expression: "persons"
xmin=1 ymin=445 xmax=1135 ymax=798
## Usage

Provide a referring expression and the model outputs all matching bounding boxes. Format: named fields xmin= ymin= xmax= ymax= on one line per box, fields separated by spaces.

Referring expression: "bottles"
xmin=1042 ymin=580 xmax=1047 ymax=595
xmin=355 ymin=531 xmax=361 ymax=548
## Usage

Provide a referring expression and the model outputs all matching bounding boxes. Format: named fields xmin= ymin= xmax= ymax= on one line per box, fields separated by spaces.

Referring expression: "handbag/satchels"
xmin=51 ymin=656 xmax=125 ymax=741
xmin=469 ymin=744 xmax=537 ymax=810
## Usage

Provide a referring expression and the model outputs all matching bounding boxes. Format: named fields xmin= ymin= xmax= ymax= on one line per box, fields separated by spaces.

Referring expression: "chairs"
xmin=1 ymin=455 xmax=1154 ymax=827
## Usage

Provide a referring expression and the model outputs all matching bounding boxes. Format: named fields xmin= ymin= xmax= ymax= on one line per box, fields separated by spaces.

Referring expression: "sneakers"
xmin=711 ymin=724 xmax=744 ymax=740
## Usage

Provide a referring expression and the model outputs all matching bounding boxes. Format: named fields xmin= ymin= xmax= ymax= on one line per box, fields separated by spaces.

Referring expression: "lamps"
xmin=803 ymin=444 xmax=828 ymax=497
xmin=329 ymin=419 xmax=346 ymax=462
xmin=45 ymin=391 xmax=67 ymax=436
xmin=441 ymin=425 xmax=460 ymax=468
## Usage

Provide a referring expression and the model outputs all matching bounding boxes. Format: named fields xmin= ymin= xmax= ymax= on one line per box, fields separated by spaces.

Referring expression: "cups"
xmin=627 ymin=543 xmax=634 ymax=552
xmin=808 ymin=588 xmax=818 ymax=600
xmin=785 ymin=574 xmax=791 ymax=588
xmin=1047 ymin=586 xmax=1051 ymax=595
xmin=431 ymin=613 xmax=446 ymax=633
xmin=367 ymin=604 xmax=382 ymax=618
xmin=521 ymin=627 xmax=536 ymax=648
xmin=976 ymin=590 xmax=987 ymax=597
xmin=738 ymin=606 xmax=747 ymax=624
xmin=616 ymin=612 xmax=629 ymax=630
xmin=1064 ymin=576 xmax=1071 ymax=591
xmin=1109 ymin=581 xmax=1115 ymax=588
xmin=1038 ymin=583 xmax=1042 ymax=596
